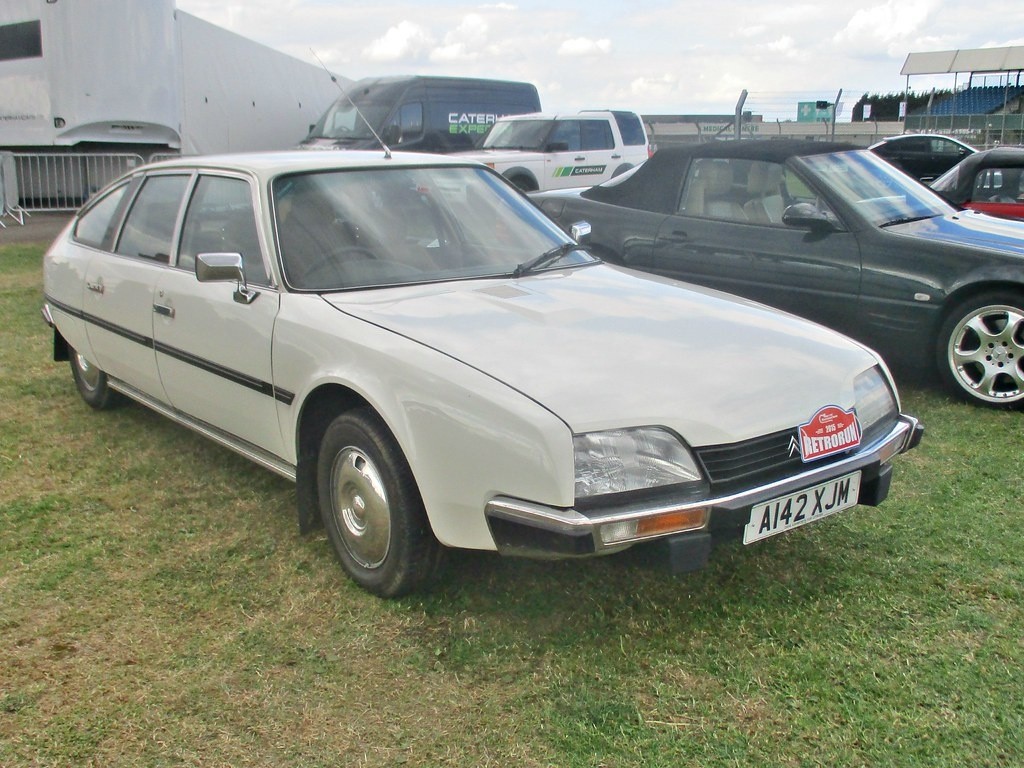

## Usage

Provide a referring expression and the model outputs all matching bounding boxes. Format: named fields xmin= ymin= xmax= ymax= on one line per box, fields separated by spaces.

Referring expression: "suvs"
xmin=442 ymin=107 xmax=656 ymax=194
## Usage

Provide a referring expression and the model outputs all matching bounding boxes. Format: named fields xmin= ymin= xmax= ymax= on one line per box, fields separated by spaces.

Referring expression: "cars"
xmin=865 ymin=134 xmax=981 ymax=186
xmin=37 ymin=149 xmax=925 ymax=601
xmin=527 ymin=138 xmax=1024 ymax=410
xmin=928 ymin=146 xmax=1024 ymax=221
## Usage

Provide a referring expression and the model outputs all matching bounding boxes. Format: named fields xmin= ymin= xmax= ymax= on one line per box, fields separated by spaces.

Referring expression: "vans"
xmin=291 ymin=74 xmax=542 ymax=155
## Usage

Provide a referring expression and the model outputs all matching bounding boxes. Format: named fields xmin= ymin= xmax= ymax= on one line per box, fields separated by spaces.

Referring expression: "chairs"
xmin=363 ymin=206 xmax=438 ymax=271
xmin=282 ymin=190 xmax=362 ymax=280
xmin=701 ymin=161 xmax=749 ymax=220
xmin=743 ymin=162 xmax=785 ymax=225
